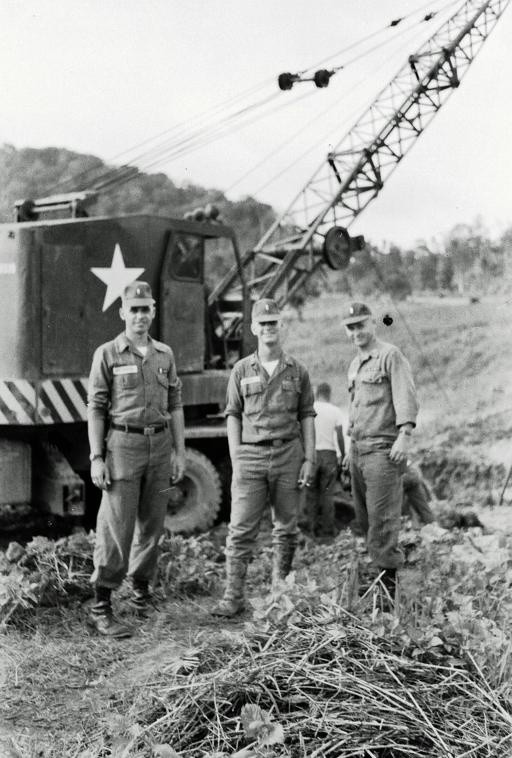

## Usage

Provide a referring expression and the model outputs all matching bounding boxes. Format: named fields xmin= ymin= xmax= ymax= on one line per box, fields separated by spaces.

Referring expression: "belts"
xmin=241 ymin=436 xmax=292 ymax=447
xmin=110 ymin=421 xmax=168 ymax=436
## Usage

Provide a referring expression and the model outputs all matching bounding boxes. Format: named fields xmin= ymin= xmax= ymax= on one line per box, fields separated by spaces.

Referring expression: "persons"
xmin=303 ymin=383 xmax=346 ymax=544
xmin=209 ymin=298 xmax=319 ymax=619
xmin=400 ymin=460 xmax=450 ymax=538
xmin=341 ymin=302 xmax=420 ymax=611
xmin=86 ymin=280 xmax=189 ymax=640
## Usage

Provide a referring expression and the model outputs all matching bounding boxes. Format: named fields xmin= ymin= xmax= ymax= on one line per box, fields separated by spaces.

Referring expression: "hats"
xmin=121 ymin=282 xmax=156 ymax=307
xmin=252 ymin=299 xmax=281 ymax=323
xmin=340 ymin=302 xmax=372 ymax=325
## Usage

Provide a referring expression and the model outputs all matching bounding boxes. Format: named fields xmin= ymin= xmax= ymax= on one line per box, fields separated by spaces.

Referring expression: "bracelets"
xmin=398 ymin=430 xmax=411 ymax=436
xmin=305 ymin=458 xmax=316 ymax=465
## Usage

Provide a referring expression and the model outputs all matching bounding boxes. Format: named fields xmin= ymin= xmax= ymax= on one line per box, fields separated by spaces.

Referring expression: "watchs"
xmin=90 ymin=453 xmax=102 ymax=461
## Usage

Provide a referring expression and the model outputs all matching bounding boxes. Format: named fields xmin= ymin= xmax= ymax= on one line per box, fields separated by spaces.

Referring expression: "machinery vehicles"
xmin=1 ymin=0 xmax=510 ymax=550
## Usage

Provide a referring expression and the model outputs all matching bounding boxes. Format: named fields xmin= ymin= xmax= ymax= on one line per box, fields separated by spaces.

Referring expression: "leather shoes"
xmin=87 ymin=602 xmax=132 ymax=637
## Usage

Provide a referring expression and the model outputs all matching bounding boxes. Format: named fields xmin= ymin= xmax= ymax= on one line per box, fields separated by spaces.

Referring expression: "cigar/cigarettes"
xmin=300 ymin=479 xmax=311 ymax=487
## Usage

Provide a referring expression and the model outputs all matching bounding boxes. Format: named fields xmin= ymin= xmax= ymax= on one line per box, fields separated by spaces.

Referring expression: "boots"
xmin=375 ymin=566 xmax=399 ymax=612
xmin=210 ymin=585 xmax=249 ymax=616
xmin=127 ymin=568 xmax=148 ymax=603
